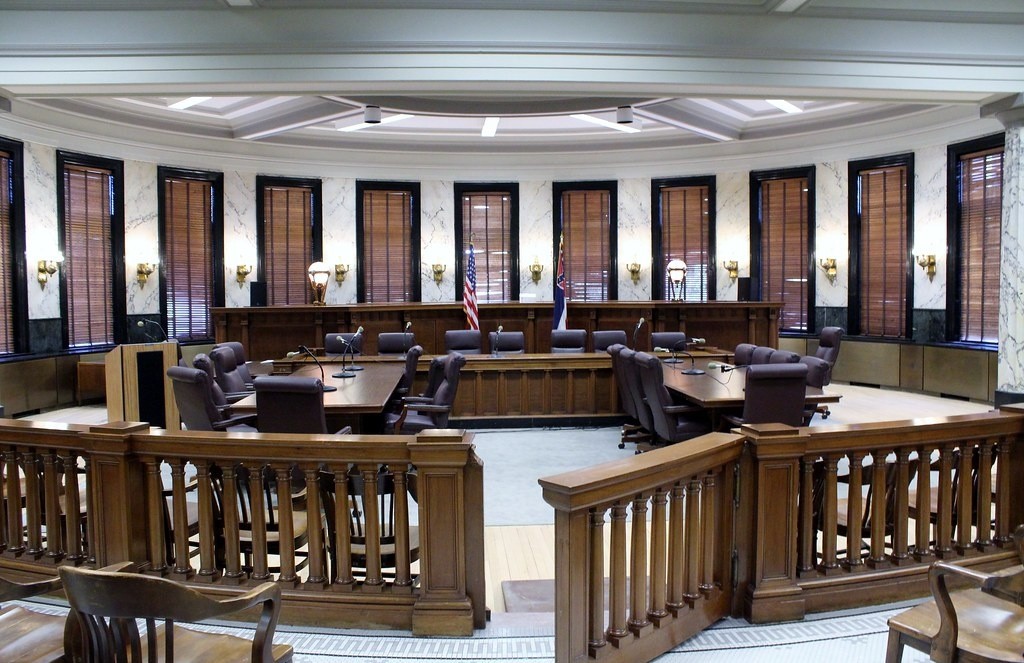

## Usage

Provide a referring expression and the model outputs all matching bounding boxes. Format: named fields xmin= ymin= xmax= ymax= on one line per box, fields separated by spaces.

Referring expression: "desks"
xmin=230 ymin=365 xmax=405 ymax=435
xmin=661 ymin=360 xmax=843 ymax=431
xmin=207 ymin=301 xmax=785 ymax=350
xmin=271 ymin=350 xmax=735 ymax=420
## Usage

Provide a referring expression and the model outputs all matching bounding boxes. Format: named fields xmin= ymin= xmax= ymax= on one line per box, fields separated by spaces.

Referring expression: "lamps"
xmin=617 ymin=106 xmax=633 ymax=123
xmin=815 ymin=259 xmax=836 ymax=286
xmin=364 ymin=105 xmax=381 ymax=123
xmin=433 ymin=264 xmax=446 ymax=286
xmin=137 ymin=263 xmax=155 ymax=289
xmin=667 ymin=259 xmax=686 ymax=301
xmin=626 ymin=263 xmax=641 ymax=285
xmin=722 ymin=260 xmax=739 ymax=281
xmin=38 ymin=251 xmax=64 ymax=291
xmin=308 ymin=261 xmax=330 ymax=306
xmin=237 ymin=265 xmax=252 ymax=287
xmin=529 ymin=264 xmax=543 ymax=286
xmin=918 ymin=255 xmax=936 ymax=283
xmin=335 ymin=264 xmax=349 ymax=288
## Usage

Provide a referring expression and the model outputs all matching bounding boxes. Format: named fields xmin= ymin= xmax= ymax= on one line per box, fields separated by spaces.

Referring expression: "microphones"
xmin=287 ymin=350 xmax=305 ymax=358
xmin=406 ymin=322 xmax=412 ymax=330
xmin=708 ymin=363 xmax=730 ymax=369
xmin=141 ymin=318 xmax=169 ymax=344
xmin=354 ymin=326 xmax=364 ymax=338
xmin=654 ymin=347 xmax=673 ymax=352
xmin=498 ymin=326 xmax=503 ymax=333
xmin=336 ymin=335 xmax=345 ymax=345
xmin=690 ymin=338 xmax=705 ymax=344
xmin=638 ymin=318 xmax=645 ymax=329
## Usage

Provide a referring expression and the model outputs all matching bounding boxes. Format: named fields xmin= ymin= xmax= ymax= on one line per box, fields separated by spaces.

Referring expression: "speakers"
xmin=250 ymin=281 xmax=268 ymax=307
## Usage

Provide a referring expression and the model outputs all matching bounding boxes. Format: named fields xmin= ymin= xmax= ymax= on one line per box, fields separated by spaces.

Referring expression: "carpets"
xmin=502 ymin=576 xmax=666 ymax=612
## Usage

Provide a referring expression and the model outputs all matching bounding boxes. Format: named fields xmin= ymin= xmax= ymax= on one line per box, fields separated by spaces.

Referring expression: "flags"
xmin=553 ymin=242 xmax=567 ymax=330
xmin=463 ymin=242 xmax=479 ymax=330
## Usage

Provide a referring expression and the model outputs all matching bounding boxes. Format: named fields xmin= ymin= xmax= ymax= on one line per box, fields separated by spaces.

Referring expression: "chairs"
xmin=0 ymin=327 xmax=996 ymax=577
xmin=58 ymin=561 xmax=292 ymax=663
xmin=0 ymin=566 xmax=93 ymax=663
xmin=883 ymin=524 xmax=1024 ymax=663
